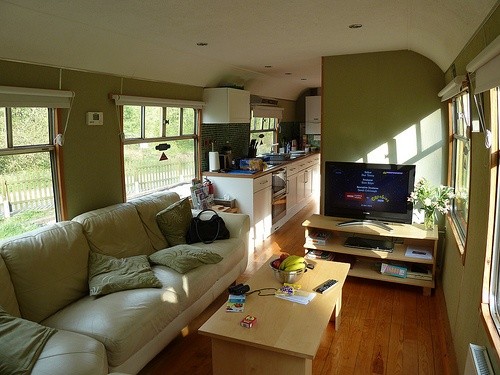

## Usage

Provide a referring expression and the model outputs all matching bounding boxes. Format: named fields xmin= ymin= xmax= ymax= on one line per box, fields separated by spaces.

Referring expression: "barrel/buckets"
xmin=221 ymin=155 xmax=232 ymax=168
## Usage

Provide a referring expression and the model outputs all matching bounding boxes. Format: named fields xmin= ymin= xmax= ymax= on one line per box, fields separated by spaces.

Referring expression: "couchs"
xmin=0 ymin=192 xmax=249 ymax=375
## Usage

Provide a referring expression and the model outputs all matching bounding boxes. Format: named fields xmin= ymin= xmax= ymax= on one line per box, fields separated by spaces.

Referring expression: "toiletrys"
xmin=287 ymin=142 xmax=291 ymax=154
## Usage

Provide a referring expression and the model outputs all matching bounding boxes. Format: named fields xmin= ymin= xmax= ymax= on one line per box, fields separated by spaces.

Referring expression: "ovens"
xmin=272 ymin=166 xmax=287 ymax=225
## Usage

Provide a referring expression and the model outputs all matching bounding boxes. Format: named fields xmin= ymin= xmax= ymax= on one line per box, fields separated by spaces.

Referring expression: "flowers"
xmin=407 ymin=177 xmax=456 ymax=228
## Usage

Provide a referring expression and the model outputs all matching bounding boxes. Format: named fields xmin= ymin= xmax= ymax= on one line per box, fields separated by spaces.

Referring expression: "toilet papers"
xmin=209 ymin=151 xmax=220 ymax=172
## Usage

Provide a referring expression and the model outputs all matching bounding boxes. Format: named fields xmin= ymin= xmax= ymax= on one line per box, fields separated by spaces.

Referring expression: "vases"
xmin=424 ymin=208 xmax=434 ymax=231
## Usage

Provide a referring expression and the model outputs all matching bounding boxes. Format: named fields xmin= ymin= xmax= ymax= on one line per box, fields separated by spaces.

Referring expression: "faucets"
xmin=269 ymin=143 xmax=279 ymax=153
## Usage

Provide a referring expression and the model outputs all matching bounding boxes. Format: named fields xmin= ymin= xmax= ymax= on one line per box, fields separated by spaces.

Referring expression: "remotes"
xmin=316 ymin=280 xmax=338 ymax=294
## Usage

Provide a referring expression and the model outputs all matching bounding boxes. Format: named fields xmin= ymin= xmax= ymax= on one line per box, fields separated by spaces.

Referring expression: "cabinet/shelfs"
xmin=301 ymin=214 xmax=438 ymax=296
xmin=305 ymin=96 xmax=321 ymax=135
xmin=203 ymin=88 xmax=251 ymax=124
xmin=200 ymin=153 xmax=320 ymax=248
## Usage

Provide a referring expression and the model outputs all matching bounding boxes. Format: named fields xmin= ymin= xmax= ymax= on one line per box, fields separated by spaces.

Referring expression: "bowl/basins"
xmin=269 ymin=258 xmax=306 ymax=284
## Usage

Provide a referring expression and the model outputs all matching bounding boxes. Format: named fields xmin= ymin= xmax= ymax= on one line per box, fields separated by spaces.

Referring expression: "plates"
xmin=291 ymin=150 xmax=304 ymax=153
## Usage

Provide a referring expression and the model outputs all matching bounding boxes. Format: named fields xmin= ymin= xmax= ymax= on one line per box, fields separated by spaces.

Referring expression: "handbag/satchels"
xmin=185 ymin=209 xmax=230 ymax=245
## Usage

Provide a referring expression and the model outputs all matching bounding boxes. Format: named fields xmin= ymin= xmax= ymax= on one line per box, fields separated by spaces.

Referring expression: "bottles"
xmin=286 ymin=142 xmax=290 ymax=154
xmin=224 ymin=141 xmax=232 ymax=154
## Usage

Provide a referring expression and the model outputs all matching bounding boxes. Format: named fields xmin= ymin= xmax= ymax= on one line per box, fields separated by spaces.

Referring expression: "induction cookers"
xmin=265 ymin=155 xmax=303 ymax=160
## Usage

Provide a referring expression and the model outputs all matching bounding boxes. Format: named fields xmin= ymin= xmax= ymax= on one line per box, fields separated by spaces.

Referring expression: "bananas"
xmin=279 ymin=256 xmax=307 ymax=272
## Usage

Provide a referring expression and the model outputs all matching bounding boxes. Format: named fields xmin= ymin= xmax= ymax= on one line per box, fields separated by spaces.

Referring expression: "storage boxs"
xmin=240 ymin=158 xmax=264 ymax=171
xmin=381 ymin=263 xmax=407 ymax=279
xmin=305 ymin=230 xmax=331 ymax=246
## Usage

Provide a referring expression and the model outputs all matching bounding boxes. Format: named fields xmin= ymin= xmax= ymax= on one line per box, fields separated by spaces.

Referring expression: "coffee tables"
xmin=198 ymin=254 xmax=350 ymax=374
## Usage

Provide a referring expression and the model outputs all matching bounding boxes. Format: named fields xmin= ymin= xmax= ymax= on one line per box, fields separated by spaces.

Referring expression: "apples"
xmin=272 ymin=254 xmax=289 ymax=268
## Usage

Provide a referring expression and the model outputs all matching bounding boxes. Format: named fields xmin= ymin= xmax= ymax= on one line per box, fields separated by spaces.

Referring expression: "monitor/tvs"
xmin=324 ymin=161 xmax=416 ymax=231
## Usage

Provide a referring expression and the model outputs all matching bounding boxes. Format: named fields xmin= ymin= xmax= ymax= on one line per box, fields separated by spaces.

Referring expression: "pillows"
xmin=88 ymin=250 xmax=162 ymax=296
xmin=148 ymin=244 xmax=223 ymax=273
xmin=0 ymin=306 xmax=58 ymax=375
xmin=156 ymin=196 xmax=193 ymax=246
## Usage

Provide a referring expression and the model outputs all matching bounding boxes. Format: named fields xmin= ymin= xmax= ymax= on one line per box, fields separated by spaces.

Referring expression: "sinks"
xmin=262 ymin=152 xmax=291 ymax=161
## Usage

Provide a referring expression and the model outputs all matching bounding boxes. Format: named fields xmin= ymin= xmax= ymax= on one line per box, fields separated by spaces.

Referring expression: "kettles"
xmin=271 ymin=143 xmax=280 ymax=153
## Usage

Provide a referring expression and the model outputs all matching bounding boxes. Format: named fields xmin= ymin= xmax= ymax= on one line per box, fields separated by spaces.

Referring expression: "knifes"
xmin=249 ymin=139 xmax=258 ymax=148
